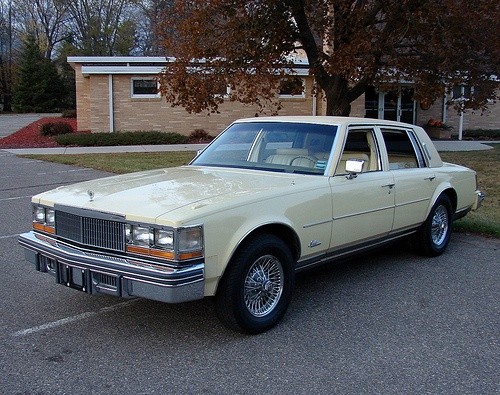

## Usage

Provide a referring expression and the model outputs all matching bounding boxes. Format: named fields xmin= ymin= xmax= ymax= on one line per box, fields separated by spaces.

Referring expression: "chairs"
xmin=264 ymin=147 xmax=319 ymax=168
xmin=336 ymin=151 xmax=371 ymax=173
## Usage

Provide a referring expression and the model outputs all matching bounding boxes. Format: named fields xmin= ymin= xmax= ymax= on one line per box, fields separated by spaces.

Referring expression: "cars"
xmin=17 ymin=115 xmax=484 ymax=337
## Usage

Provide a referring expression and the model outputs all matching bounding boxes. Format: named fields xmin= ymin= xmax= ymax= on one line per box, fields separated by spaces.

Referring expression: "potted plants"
xmin=425 ymin=118 xmax=455 ymax=140
